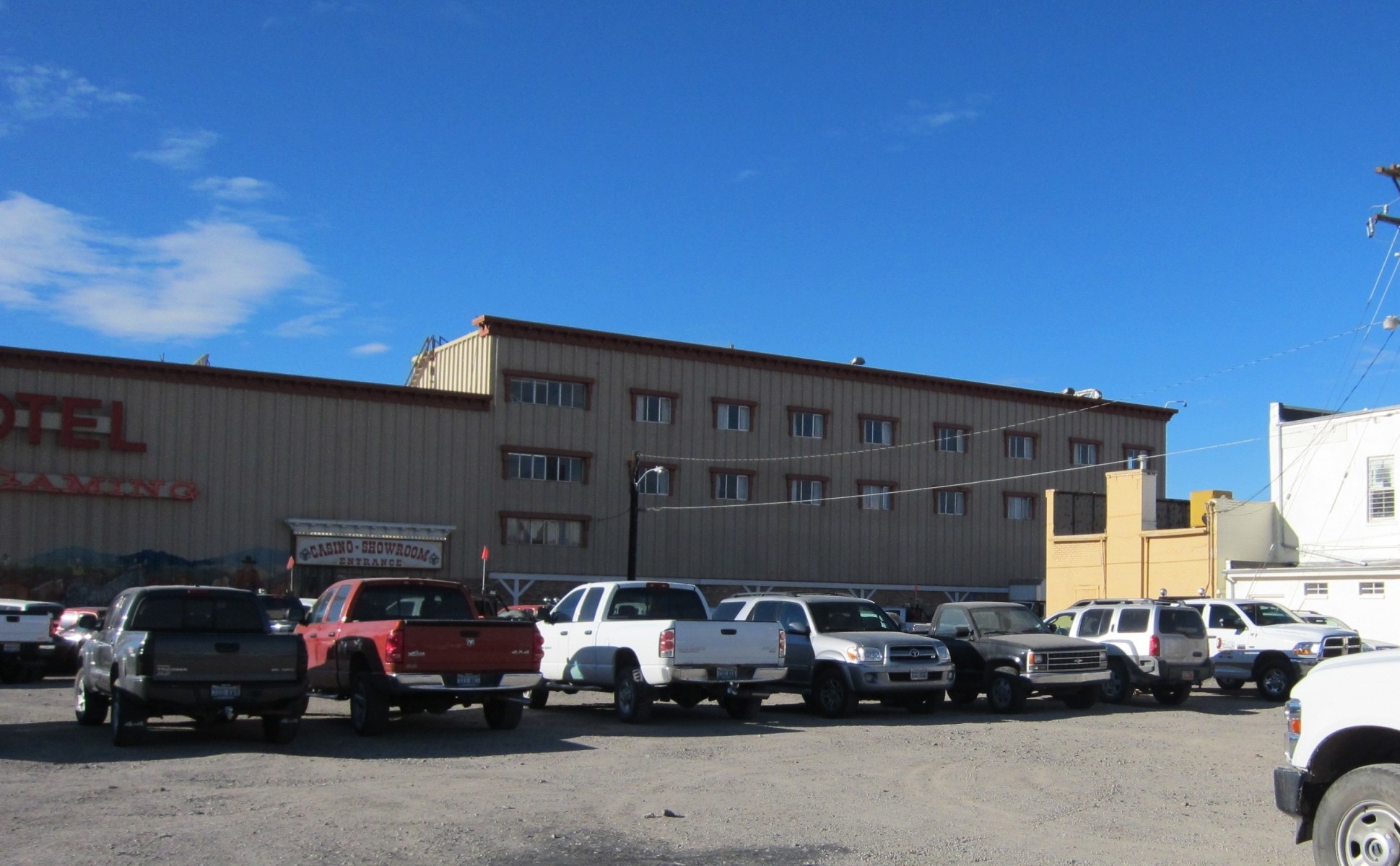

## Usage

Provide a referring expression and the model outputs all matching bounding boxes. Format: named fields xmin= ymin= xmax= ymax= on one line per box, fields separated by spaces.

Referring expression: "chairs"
xmin=618 ymin=605 xmax=636 ymax=615
xmin=426 ymin=601 xmax=455 ymax=620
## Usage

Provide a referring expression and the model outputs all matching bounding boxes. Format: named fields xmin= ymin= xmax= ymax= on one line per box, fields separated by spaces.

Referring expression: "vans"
xmin=0 ymin=598 xmax=64 ymax=644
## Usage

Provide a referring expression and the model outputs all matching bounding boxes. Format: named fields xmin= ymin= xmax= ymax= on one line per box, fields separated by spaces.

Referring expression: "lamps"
xmin=1166 ymin=400 xmax=1187 ymax=409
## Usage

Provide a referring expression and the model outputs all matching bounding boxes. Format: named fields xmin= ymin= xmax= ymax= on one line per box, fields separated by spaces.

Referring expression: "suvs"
xmin=1037 ymin=599 xmax=1214 ymax=707
xmin=256 ymin=591 xmax=306 ymax=635
xmin=712 ymin=592 xmax=955 ymax=719
xmin=1160 ymin=589 xmax=1361 ymax=701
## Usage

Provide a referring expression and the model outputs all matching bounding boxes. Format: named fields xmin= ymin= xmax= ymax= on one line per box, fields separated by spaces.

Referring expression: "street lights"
xmin=628 ymin=465 xmax=666 ymax=580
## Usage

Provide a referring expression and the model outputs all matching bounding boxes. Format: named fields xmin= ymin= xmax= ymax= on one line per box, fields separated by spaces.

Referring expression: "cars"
xmin=1298 ymin=614 xmax=1400 ymax=652
xmin=54 ymin=607 xmax=109 ymax=676
xmin=1272 ymin=648 xmax=1400 ymax=866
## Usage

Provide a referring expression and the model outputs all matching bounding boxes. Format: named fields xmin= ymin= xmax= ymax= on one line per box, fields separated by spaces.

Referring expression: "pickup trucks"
xmin=522 ymin=581 xmax=788 ymax=723
xmin=899 ymin=602 xmax=1112 ymax=714
xmin=292 ymin=576 xmax=545 ymax=734
xmin=73 ymin=585 xmax=310 ymax=745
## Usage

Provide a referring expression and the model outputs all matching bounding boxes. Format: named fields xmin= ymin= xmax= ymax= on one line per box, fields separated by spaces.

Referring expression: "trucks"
xmin=0 ymin=611 xmax=54 ymax=669
